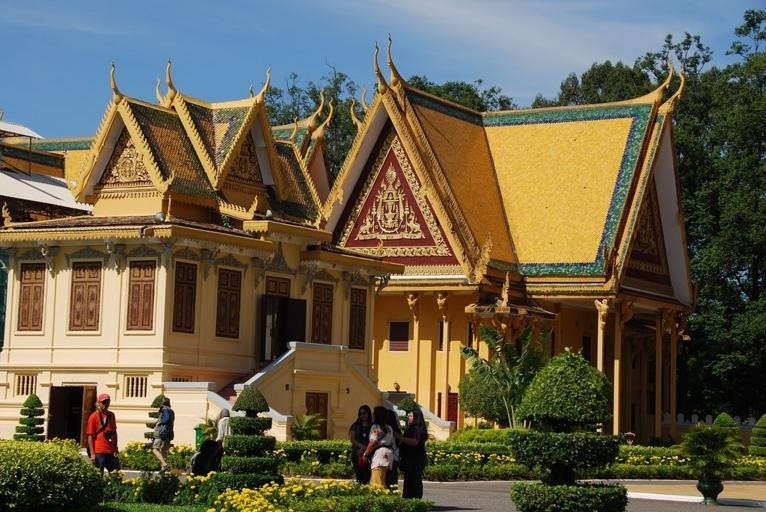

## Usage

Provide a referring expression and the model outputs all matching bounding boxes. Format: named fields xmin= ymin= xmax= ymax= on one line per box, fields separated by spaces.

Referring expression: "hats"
xmin=98 ymin=393 xmax=110 ymax=403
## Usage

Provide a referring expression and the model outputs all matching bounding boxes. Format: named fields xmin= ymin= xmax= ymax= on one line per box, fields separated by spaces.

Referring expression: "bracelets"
xmin=363 ymin=451 xmax=369 ymax=457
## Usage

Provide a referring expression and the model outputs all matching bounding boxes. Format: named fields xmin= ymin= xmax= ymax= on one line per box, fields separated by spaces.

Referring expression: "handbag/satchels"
xmin=153 ymin=426 xmax=170 ymax=439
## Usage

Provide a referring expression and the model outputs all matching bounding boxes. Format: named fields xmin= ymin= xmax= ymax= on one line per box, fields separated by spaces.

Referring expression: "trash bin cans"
xmin=193 ymin=424 xmax=205 ymax=451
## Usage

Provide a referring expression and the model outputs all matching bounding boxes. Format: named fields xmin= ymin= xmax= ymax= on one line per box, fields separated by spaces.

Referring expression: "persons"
xmin=215 ymin=409 xmax=231 ymax=450
xmin=394 ymin=410 xmax=428 ymax=496
xmin=362 ymin=404 xmax=395 ymax=488
xmin=349 ymin=404 xmax=374 ymax=484
xmin=86 ymin=393 xmax=121 ymax=477
xmin=152 ymin=397 xmax=174 ymax=474
xmin=386 ymin=410 xmax=403 ymax=488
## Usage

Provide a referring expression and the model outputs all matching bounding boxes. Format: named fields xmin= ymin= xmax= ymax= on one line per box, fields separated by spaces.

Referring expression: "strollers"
xmin=188 ymin=439 xmax=224 ymax=474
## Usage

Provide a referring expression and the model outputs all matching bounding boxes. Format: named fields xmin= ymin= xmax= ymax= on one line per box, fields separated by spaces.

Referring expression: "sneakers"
xmin=158 ymin=464 xmax=171 ymax=475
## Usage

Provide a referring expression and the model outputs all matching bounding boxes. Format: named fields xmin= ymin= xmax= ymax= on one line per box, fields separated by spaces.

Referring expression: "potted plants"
xmin=679 ymin=426 xmax=738 ymax=505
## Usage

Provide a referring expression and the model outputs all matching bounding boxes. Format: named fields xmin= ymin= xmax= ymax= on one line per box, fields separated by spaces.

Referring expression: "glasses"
xmin=359 ymin=411 xmax=367 ymax=416
